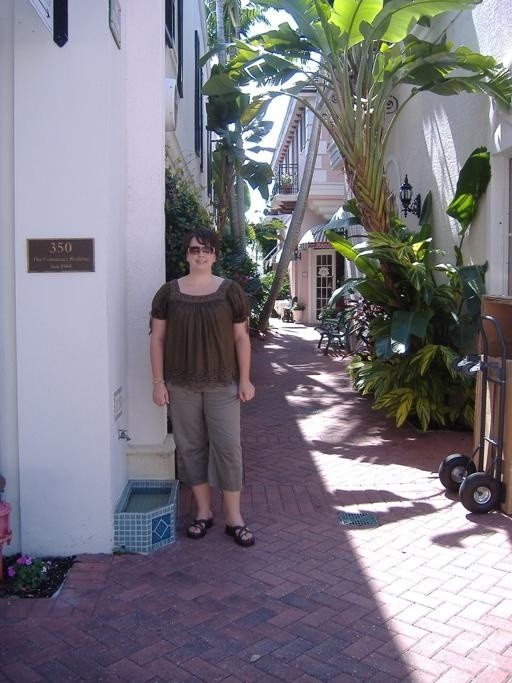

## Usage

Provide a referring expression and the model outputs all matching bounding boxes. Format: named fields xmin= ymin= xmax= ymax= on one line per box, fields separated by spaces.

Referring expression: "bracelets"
xmin=151 ymin=380 xmax=166 ymax=384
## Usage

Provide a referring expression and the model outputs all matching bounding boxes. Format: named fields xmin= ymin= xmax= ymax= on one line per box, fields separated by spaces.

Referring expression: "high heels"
xmin=187 ymin=517 xmax=213 ymax=539
xmin=225 ymin=523 xmax=255 ymax=547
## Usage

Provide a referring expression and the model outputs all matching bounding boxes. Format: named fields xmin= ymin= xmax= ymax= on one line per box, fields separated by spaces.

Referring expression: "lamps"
xmin=398 ymin=174 xmax=421 ymax=218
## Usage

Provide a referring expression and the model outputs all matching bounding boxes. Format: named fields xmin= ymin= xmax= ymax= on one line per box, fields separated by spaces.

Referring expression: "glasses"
xmin=188 ymin=246 xmax=213 ymax=255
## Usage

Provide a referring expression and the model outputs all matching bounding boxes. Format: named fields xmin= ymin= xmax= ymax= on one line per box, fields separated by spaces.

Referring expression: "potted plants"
xmin=279 ymin=172 xmax=294 ymax=193
xmin=291 ymin=301 xmax=306 ymax=324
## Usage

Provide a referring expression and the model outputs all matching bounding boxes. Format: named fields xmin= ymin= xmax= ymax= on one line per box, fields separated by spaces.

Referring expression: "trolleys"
xmin=439 ymin=315 xmax=512 ymax=518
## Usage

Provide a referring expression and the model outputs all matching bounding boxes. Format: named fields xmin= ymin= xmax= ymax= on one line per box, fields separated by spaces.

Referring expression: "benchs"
xmin=315 ymin=314 xmax=347 ymax=355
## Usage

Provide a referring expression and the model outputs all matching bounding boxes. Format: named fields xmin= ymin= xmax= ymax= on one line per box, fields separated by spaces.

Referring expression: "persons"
xmin=150 ymin=226 xmax=256 ymax=547
